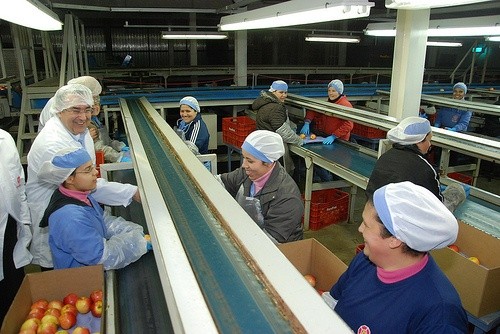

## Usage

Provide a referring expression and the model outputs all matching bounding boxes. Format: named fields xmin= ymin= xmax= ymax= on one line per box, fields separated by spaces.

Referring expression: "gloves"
xmin=120 ymin=152 xmax=131 ymax=163
xmin=446 ymin=126 xmax=456 ymax=131
xmin=122 ymin=147 xmax=130 ymax=151
xmin=439 ymin=185 xmax=446 ymax=193
xmin=434 ymin=123 xmax=438 ymax=127
xmin=464 ymin=186 xmax=470 ymax=198
xmin=300 ymin=123 xmax=310 ymax=137
xmin=322 ymin=135 xmax=336 ymax=146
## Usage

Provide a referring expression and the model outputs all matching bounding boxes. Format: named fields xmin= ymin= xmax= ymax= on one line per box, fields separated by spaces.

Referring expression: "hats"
xmin=180 ymin=96 xmax=200 ymax=114
xmin=38 ymin=147 xmax=92 ymax=186
xmin=372 ymin=180 xmax=458 ymax=252
xmin=241 ymin=130 xmax=285 ymax=165
xmin=387 ymin=117 xmax=431 ymax=145
xmin=454 ymin=82 xmax=467 ymax=94
xmin=328 ymin=79 xmax=343 ymax=96
xmin=268 ymin=80 xmax=287 ymax=92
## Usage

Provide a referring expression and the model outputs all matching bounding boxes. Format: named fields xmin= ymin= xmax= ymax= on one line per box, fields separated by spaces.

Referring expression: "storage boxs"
xmin=276 ymin=239 xmax=348 ymax=295
xmin=0 ymin=263 xmax=108 ymax=334
xmin=300 ymin=190 xmax=352 ymax=231
xmin=95 ymin=152 xmax=106 ymax=178
xmin=223 ymin=116 xmax=259 ymax=148
xmin=451 ymin=172 xmax=474 ymax=184
xmin=353 ymin=124 xmax=384 ymax=138
xmin=425 ymin=219 xmax=500 ymax=317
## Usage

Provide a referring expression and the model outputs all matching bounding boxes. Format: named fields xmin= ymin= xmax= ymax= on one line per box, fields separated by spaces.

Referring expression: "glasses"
xmin=66 ymin=108 xmax=94 ymax=116
xmin=72 ymin=162 xmax=95 ymax=174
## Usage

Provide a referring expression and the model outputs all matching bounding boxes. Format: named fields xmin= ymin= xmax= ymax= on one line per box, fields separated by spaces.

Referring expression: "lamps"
xmin=0 ymin=0 xmax=65 ymax=31
xmin=161 ymin=0 xmax=500 ymax=49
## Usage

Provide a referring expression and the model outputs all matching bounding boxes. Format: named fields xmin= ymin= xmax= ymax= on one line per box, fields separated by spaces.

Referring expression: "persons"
xmin=0 ymin=128 xmax=33 ymax=327
xmin=214 ymin=130 xmax=305 ymax=243
xmin=300 ymin=79 xmax=354 ymax=181
xmin=252 ymin=81 xmax=303 ymax=173
xmin=174 ymin=96 xmax=210 ymax=163
xmin=25 ymin=84 xmax=141 ymax=271
xmin=38 ymin=76 xmax=131 ymax=163
xmin=365 ymin=117 xmax=466 ymax=213
xmin=329 ymin=181 xmax=469 ymax=334
xmin=38 ymin=147 xmax=152 ymax=271
xmin=434 ymin=82 xmax=472 ymax=132
